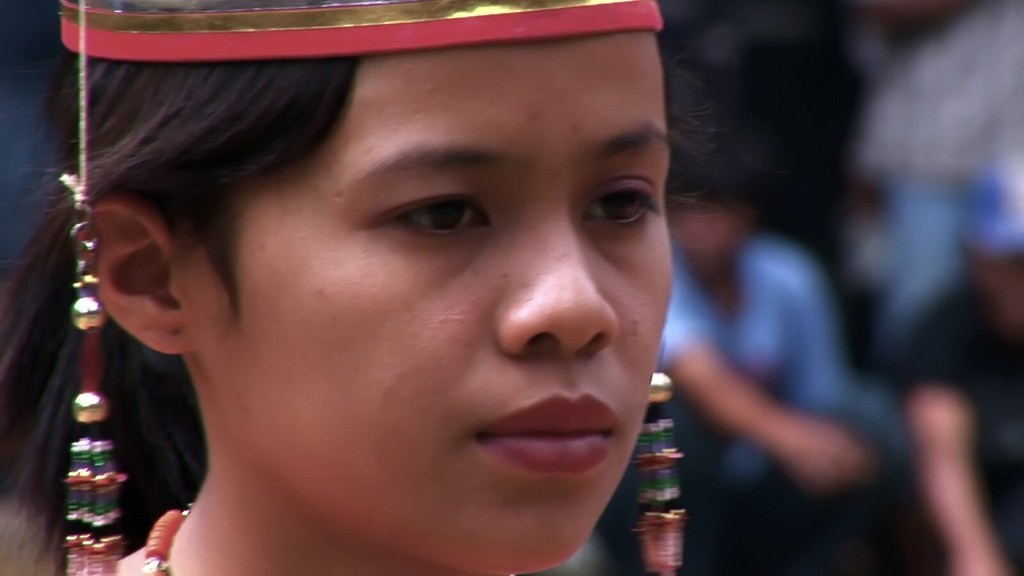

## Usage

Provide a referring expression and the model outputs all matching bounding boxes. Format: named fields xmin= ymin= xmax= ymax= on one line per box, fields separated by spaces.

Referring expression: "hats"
xmin=59 ymin=0 xmax=686 ymax=576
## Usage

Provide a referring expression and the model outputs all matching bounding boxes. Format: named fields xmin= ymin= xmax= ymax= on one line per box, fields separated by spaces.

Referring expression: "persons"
xmin=0 ymin=1 xmax=673 ymax=575
xmin=576 ymin=2 xmax=1023 ymax=575
